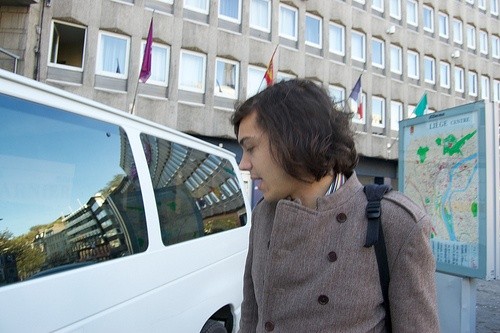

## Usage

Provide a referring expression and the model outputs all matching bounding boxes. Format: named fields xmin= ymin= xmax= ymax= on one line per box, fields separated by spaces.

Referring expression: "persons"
xmin=230 ymin=77 xmax=440 ymax=333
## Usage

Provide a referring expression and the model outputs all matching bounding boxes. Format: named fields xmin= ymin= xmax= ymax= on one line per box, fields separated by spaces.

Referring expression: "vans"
xmin=0 ymin=66 xmax=254 ymax=333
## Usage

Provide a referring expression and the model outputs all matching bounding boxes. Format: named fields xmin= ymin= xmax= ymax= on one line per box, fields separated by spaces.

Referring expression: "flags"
xmin=347 ymin=78 xmax=363 ymax=121
xmin=138 ymin=17 xmax=153 ymax=83
xmin=263 ymin=52 xmax=273 ymax=88
xmin=212 ymin=179 xmax=223 ymax=199
xmin=131 ymin=144 xmax=151 ymax=180
xmin=412 ymin=91 xmax=428 ymax=117
